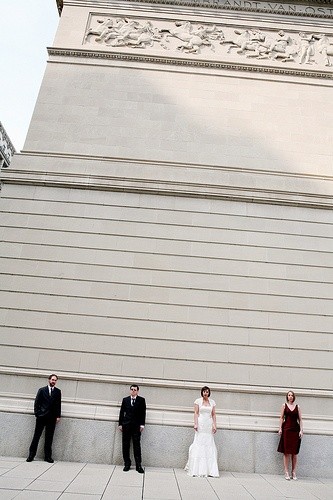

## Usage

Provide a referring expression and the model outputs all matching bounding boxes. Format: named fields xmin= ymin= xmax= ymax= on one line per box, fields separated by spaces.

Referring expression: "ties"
xmin=50 ymin=388 xmax=52 ymax=397
xmin=132 ymin=399 xmax=135 ymax=406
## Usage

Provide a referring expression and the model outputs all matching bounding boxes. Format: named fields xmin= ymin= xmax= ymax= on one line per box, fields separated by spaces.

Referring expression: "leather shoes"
xmin=123 ymin=466 xmax=130 ymax=471
xmin=136 ymin=465 xmax=144 ymax=473
xmin=44 ymin=454 xmax=54 ymax=463
xmin=26 ymin=452 xmax=36 ymax=463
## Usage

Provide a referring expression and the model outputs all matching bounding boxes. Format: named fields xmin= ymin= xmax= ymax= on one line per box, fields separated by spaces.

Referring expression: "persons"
xmin=119 ymin=385 xmax=146 ymax=473
xmin=277 ymin=391 xmax=303 ymax=480
xmin=27 ymin=375 xmax=61 ymax=463
xmin=185 ymin=387 xmax=219 ymax=478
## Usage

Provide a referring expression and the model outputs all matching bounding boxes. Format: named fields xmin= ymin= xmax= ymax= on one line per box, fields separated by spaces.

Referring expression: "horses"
xmin=86 ymin=16 xmax=298 ymax=63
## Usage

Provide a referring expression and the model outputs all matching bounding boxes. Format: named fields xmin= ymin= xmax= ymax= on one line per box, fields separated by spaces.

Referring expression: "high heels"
xmin=284 ymin=472 xmax=291 ymax=480
xmin=292 ymin=471 xmax=297 ymax=480
